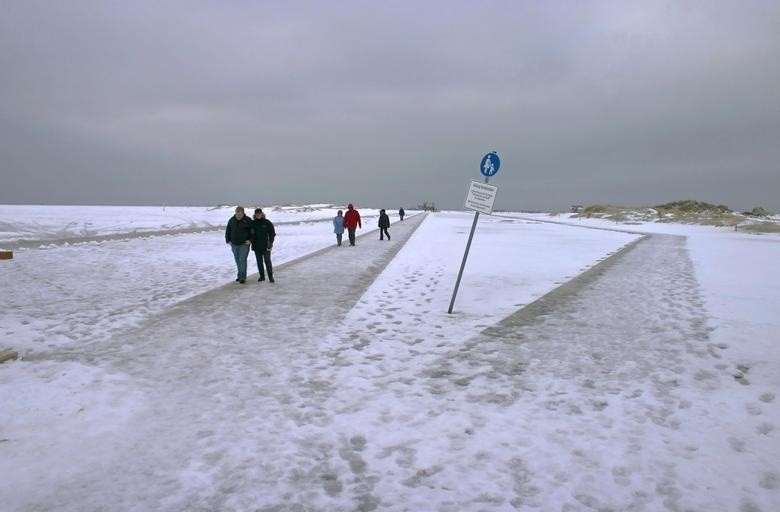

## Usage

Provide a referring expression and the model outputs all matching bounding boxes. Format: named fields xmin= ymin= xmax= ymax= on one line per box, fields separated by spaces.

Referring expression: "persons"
xmin=250 ymin=209 xmax=276 ymax=283
xmin=225 ymin=207 xmax=252 ymax=283
xmin=399 ymin=206 xmax=405 ymax=220
xmin=344 ymin=204 xmax=361 ymax=247
xmin=333 ymin=210 xmax=344 ymax=246
xmin=378 ymin=209 xmax=391 ymax=241
